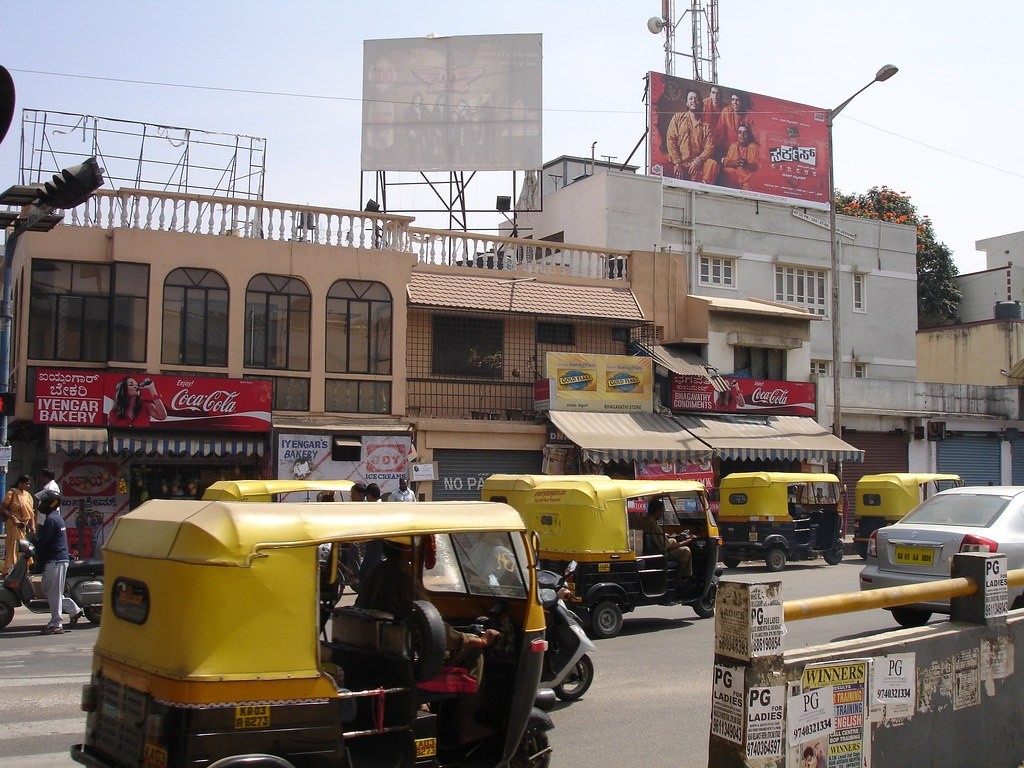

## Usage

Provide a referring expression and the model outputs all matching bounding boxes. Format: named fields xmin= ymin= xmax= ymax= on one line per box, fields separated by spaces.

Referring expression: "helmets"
xmin=34 ymin=489 xmax=61 ymax=514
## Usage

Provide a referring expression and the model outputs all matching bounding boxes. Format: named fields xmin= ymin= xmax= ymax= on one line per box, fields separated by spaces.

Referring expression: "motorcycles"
xmin=198 ymin=474 xmax=370 ymax=595
xmin=854 ymin=470 xmax=966 ymax=560
xmin=716 ymin=472 xmax=845 ymax=572
xmin=480 ymin=473 xmax=725 ymax=639
xmin=70 ymin=496 xmax=560 ymax=768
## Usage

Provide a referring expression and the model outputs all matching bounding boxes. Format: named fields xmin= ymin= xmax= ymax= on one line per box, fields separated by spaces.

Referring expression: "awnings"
xmin=46 ymin=427 xmax=265 ymax=458
xmin=544 ymin=412 xmax=715 ymax=463
xmin=673 ymin=412 xmax=864 ymax=463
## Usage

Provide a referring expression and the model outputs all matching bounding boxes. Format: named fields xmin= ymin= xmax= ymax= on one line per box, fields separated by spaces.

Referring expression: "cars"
xmin=856 ymin=485 xmax=1024 ymax=627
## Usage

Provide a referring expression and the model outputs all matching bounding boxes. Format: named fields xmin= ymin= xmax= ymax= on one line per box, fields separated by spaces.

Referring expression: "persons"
xmin=702 ymin=85 xmax=728 ymax=130
xmin=387 ymin=477 xmax=416 ymax=502
xmin=634 ymin=500 xmax=696 ymax=582
xmin=666 ymin=91 xmax=719 ymax=184
xmin=723 ymin=124 xmax=761 ymax=190
xmin=0 ymin=474 xmax=35 ymax=575
xmin=24 ymin=490 xmax=83 ymax=634
xmin=713 ymin=89 xmax=761 ymax=150
xmin=322 ymin=483 xmax=383 ymax=578
xmin=356 ymin=555 xmax=500 ymax=686
xmin=108 ymin=377 xmax=166 ymax=427
xmin=30 ymin=468 xmax=61 ymax=570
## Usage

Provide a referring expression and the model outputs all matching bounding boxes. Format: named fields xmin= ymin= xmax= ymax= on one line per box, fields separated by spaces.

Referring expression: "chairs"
xmin=786 ymin=502 xmax=812 ymax=545
xmin=640 ymin=535 xmax=679 ymax=597
xmin=330 ymin=607 xmax=427 ymax=720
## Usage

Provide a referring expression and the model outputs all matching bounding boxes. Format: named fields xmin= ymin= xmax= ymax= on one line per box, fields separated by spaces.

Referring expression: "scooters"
xmin=490 ymin=534 xmax=596 ymax=703
xmin=0 ymin=521 xmax=105 ymax=630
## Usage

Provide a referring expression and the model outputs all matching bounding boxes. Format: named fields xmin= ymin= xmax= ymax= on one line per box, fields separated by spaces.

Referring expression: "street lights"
xmin=823 ymin=63 xmax=901 ymax=494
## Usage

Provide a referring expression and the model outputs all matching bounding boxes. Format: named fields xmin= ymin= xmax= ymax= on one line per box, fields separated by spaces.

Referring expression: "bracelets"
xmin=153 ymin=394 xmax=162 ymax=400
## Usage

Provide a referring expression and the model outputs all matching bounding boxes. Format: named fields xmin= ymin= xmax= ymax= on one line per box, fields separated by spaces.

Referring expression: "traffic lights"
xmin=0 ymin=393 xmax=18 ymax=416
xmin=36 ymin=160 xmax=105 ymax=209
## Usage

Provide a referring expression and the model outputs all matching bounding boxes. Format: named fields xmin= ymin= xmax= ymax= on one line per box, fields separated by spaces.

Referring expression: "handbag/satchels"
xmin=0 ymin=490 xmax=15 ymax=522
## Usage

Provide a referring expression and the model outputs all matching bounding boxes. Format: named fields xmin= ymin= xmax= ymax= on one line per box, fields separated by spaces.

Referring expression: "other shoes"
xmin=455 ymin=725 xmax=505 ymax=747
xmin=40 ymin=626 xmax=64 ymax=635
xmin=69 ymin=610 xmax=83 ymax=629
